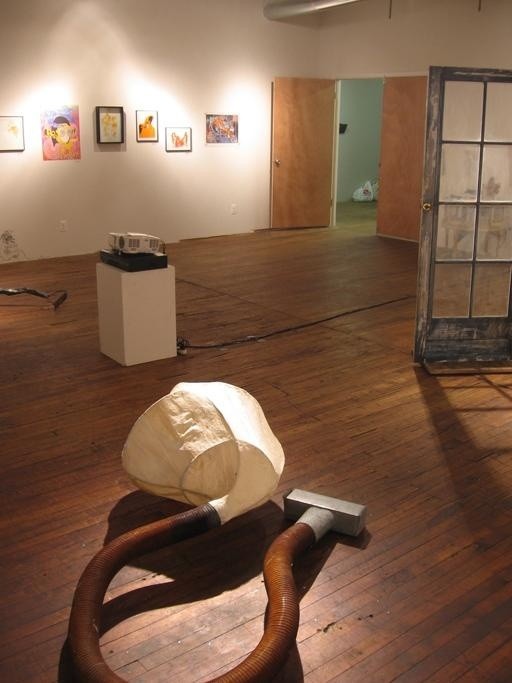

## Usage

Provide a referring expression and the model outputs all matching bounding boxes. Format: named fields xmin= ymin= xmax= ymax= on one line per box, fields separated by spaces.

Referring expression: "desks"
xmin=441 ymin=214 xmax=510 ymax=259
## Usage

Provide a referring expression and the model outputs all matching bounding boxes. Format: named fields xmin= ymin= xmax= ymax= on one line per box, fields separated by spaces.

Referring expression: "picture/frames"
xmin=136 ymin=109 xmax=158 ymax=142
xmin=0 ymin=116 xmax=25 ymax=151
xmin=96 ymin=106 xmax=124 ymax=144
xmin=165 ymin=127 xmax=192 ymax=151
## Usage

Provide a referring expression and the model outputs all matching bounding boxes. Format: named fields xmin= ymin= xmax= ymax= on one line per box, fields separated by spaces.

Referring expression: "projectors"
xmin=108 ymin=232 xmax=160 ymax=253
